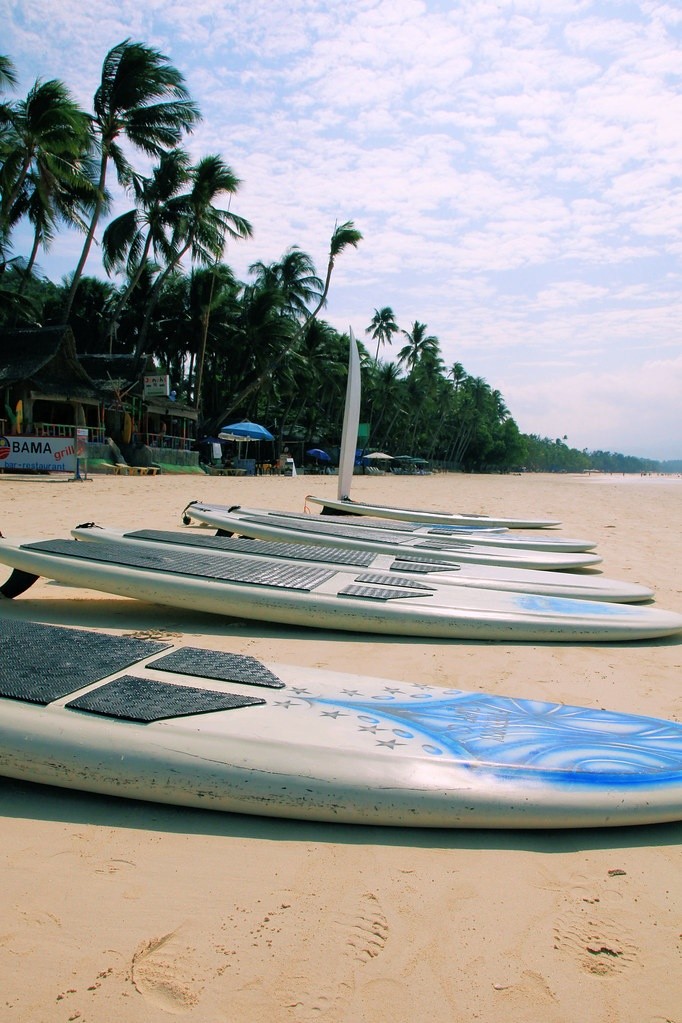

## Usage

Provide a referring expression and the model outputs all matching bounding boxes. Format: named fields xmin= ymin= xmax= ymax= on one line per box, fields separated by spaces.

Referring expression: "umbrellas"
xmin=364 ymin=452 xmax=394 ymax=459
xmin=394 ymin=455 xmax=428 ymax=464
xmin=197 ymin=436 xmax=223 ymax=444
xmin=308 ymin=448 xmax=330 ymax=461
xmin=218 ymin=420 xmax=275 ymax=459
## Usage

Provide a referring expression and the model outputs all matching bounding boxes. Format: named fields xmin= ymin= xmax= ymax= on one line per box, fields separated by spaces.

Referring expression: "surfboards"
xmin=0 ymin=612 xmax=681 ymax=829
xmin=68 ymin=521 xmax=654 ymax=602
xmin=181 ymin=495 xmax=601 ymax=567
xmin=1 ymin=541 xmax=681 ymax=640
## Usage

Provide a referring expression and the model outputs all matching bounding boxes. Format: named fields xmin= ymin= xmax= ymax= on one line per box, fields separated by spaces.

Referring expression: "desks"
xmin=254 ymin=464 xmax=272 ymax=476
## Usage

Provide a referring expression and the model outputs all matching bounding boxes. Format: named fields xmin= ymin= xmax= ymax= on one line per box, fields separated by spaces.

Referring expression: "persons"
xmin=156 ymin=419 xmax=166 ymax=445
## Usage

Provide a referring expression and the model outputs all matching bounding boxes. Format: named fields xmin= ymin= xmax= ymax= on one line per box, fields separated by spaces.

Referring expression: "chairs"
xmin=116 ymin=463 xmax=148 ymax=476
xmin=201 ymin=463 xmax=247 ymax=476
xmin=366 ymin=467 xmax=385 ymax=475
xmin=263 ymin=460 xmax=277 ymax=477
xmin=147 ymin=467 xmax=160 ymax=476
xmin=391 ymin=468 xmax=405 ymax=475
xmin=101 ymin=462 xmax=130 ymax=477
